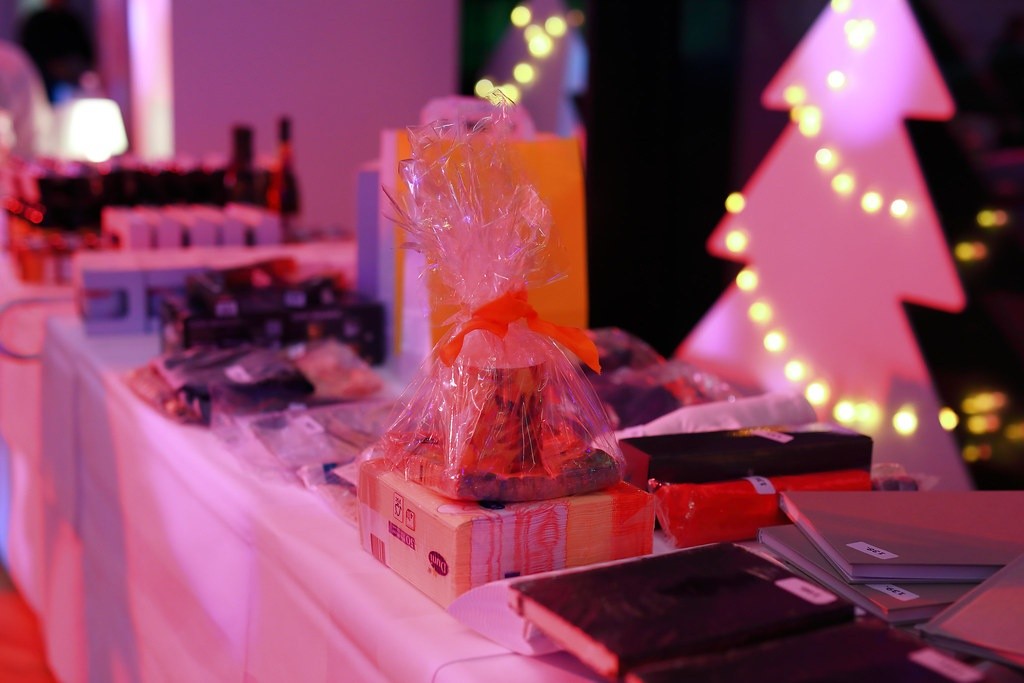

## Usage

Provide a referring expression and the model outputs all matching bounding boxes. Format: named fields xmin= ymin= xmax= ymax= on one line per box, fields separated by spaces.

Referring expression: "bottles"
xmin=266 ymin=119 xmax=300 ymax=217
xmin=224 ymin=130 xmax=255 ymax=204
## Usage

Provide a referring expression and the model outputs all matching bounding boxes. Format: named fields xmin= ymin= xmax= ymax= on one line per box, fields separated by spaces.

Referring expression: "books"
xmin=507 ymin=489 xmax=1023 ymax=683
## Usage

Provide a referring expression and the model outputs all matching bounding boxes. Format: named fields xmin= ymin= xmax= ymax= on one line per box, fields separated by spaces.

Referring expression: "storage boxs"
xmin=618 ymin=422 xmax=874 ymax=533
xmin=71 ymin=203 xmax=357 ymax=336
xmin=159 ymin=290 xmax=388 ymax=365
xmin=356 ymin=458 xmax=657 ymax=609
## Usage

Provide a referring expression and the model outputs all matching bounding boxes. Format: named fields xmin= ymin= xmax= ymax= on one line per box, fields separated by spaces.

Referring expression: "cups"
xmin=459 ymin=356 xmax=546 ymax=471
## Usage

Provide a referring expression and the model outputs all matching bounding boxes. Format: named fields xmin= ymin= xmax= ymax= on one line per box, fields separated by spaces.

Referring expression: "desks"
xmin=0 ymin=251 xmax=775 ymax=683
